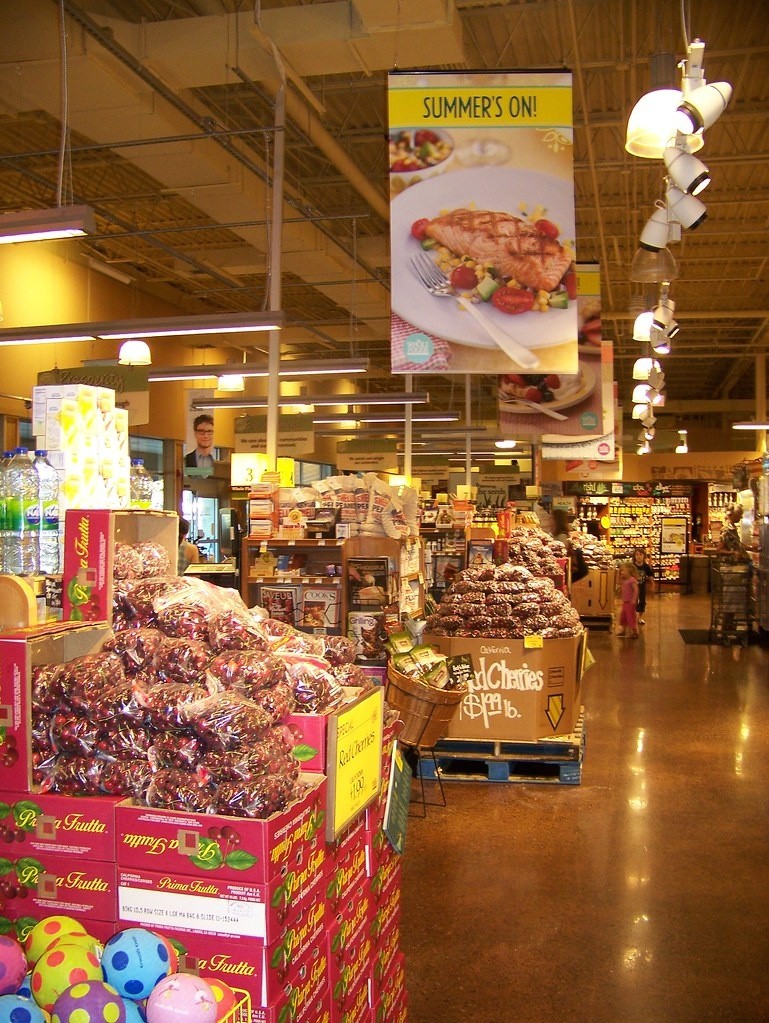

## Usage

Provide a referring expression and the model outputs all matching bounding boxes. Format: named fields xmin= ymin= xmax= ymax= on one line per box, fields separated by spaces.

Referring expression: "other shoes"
xmin=638 ymin=619 xmax=645 ymax=625
xmin=721 ymin=628 xmax=740 ymax=638
xmin=626 ymin=633 xmax=639 ymax=639
xmin=615 ymin=630 xmax=626 ymax=636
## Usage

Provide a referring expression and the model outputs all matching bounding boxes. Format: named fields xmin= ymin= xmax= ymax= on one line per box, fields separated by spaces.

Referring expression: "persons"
xmin=549 ymin=509 xmax=573 ymax=602
xmin=178 ymin=516 xmax=200 ymax=565
xmin=615 ymin=563 xmax=640 ymax=638
xmin=631 ymin=549 xmax=654 ymax=625
xmin=716 ymin=502 xmax=753 ymax=638
xmin=587 ymin=503 xmax=611 ymax=544
xmin=184 ymin=415 xmax=214 ymax=467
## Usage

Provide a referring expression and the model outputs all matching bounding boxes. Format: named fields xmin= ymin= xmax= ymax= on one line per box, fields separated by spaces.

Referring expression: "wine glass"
xmin=455 ymin=75 xmax=510 ymax=168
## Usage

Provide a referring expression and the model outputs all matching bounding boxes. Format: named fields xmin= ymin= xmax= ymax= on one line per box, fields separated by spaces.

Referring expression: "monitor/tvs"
xmin=218 ymin=508 xmax=240 ymax=555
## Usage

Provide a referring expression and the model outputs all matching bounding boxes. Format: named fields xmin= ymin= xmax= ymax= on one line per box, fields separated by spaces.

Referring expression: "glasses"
xmin=195 ymin=428 xmax=214 ymax=436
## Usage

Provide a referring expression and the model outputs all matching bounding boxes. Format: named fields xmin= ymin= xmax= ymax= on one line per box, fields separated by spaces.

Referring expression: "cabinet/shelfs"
xmin=597 ymin=507 xmax=655 ymax=591
xmin=418 ymin=527 xmax=468 ymax=600
xmin=237 ymin=537 xmax=348 ymax=637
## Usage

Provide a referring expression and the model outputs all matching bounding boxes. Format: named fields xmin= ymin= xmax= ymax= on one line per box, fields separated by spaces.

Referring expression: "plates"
xmin=390 ymin=166 xmax=577 ymax=350
xmin=498 ymin=361 xmax=595 ymax=417
xmin=543 ymin=382 xmax=614 ymax=444
xmin=578 ymin=340 xmax=601 ymax=356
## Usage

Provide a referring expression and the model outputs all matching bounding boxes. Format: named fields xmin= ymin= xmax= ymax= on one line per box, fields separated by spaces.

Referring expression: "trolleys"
xmin=706 ymin=551 xmax=759 ymax=647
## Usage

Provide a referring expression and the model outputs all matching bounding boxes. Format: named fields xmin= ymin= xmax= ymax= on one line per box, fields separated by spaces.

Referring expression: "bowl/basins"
xmin=390 ymin=129 xmax=454 ymax=192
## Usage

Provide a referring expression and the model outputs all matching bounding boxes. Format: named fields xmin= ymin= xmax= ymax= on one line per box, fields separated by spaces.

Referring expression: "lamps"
xmin=632 ymin=39 xmax=733 ymax=452
xmin=0 ymin=0 xmax=537 ymax=463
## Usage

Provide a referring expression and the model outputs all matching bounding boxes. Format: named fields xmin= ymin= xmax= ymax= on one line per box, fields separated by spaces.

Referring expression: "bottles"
xmin=131 ymin=457 xmax=152 ymax=509
xmin=0 ymin=448 xmax=59 ymax=575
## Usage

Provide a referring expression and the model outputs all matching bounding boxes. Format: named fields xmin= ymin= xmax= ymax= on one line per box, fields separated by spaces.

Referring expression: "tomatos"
xmin=410 ymin=214 xmax=577 ymax=315
xmin=390 ymin=129 xmax=439 ymax=172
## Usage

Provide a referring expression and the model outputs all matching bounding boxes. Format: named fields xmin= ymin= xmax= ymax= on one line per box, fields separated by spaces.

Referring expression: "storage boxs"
xmin=0 ymin=506 xmax=619 ymax=1023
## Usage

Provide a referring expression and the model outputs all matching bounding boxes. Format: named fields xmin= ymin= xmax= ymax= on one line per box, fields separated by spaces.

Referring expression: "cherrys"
xmin=276 ymin=797 xmax=386 ymax=1011
xmin=0 ymin=732 xmax=243 ymax=899
xmin=426 ymin=528 xmax=582 ymax=639
xmin=32 ymin=544 xmax=380 ymax=818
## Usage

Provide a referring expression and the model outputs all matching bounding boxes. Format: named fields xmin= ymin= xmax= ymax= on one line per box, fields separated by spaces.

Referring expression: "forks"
xmin=410 ymin=251 xmax=542 ymax=370
xmin=499 ymin=387 xmax=568 ymax=422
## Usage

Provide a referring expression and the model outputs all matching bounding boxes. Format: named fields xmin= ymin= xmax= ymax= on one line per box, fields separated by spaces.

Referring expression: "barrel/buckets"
xmin=383 ymin=660 xmax=469 ymax=749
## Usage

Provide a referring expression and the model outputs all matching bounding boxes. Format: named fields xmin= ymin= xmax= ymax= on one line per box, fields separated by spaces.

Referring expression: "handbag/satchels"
xmin=644 ymin=577 xmax=656 ymax=594
xmin=566 ymin=544 xmax=589 ymax=584
xmin=720 ymin=530 xmax=750 ymax=565
